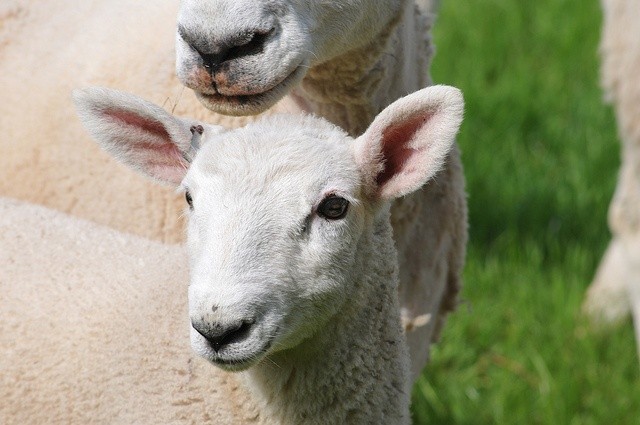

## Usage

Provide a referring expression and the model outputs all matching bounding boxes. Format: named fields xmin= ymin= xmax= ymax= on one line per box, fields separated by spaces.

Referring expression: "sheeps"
xmin=0 ymin=84 xmax=464 ymax=425
xmin=1 ymin=0 xmax=468 ymax=385
xmin=578 ymin=0 xmax=640 ymax=355
